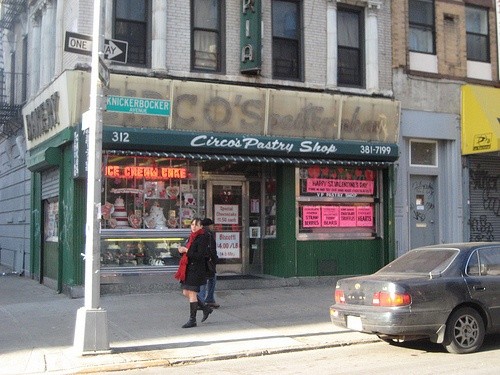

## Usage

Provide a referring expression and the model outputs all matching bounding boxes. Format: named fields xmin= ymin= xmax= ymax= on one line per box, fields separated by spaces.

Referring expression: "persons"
xmin=197 ymin=218 xmax=220 ymax=310
xmin=178 ymin=218 xmax=213 ymax=328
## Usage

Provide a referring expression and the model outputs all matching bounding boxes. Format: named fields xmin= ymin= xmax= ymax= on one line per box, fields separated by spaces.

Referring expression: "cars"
xmin=329 ymin=242 xmax=500 ymax=354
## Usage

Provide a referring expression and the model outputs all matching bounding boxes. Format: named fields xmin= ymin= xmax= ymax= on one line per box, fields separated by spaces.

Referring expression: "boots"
xmin=197 ymin=296 xmax=213 ymax=322
xmin=182 ymin=302 xmax=198 ymax=328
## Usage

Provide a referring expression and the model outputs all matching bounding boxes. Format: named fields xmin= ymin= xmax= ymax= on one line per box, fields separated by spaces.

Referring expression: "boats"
xmin=64 ymin=31 xmax=128 ymax=64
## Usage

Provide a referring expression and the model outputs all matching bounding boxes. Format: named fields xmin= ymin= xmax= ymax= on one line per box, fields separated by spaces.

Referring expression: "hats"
xmin=201 ymin=218 xmax=214 ymax=226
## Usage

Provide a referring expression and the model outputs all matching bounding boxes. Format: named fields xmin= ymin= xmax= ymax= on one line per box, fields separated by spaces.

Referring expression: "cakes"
xmin=150 ymin=259 xmax=162 ymax=265
xmin=150 ymin=201 xmax=166 ymax=227
xmin=159 ymin=252 xmax=171 ymax=258
xmin=111 ymin=197 xmax=129 ymax=225
xmin=156 ymin=242 xmax=182 ymax=248
xmin=108 ymin=244 xmax=119 ymax=249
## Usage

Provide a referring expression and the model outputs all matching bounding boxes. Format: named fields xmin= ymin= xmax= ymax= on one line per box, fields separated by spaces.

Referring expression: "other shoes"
xmin=197 ymin=306 xmax=203 ymax=310
xmin=206 ymin=302 xmax=219 ymax=308
